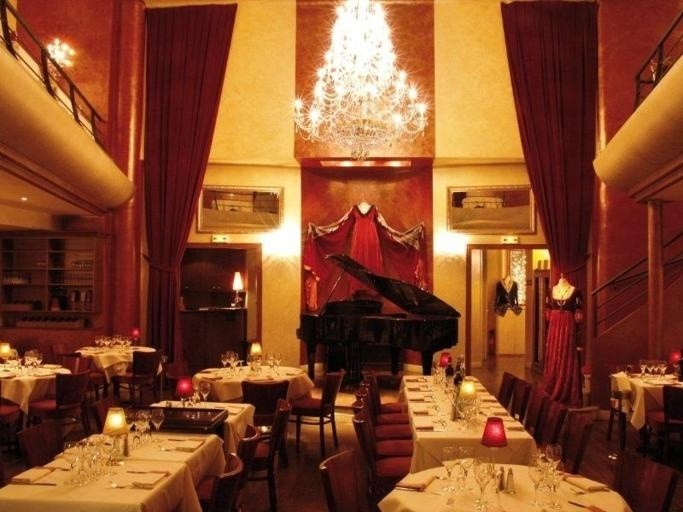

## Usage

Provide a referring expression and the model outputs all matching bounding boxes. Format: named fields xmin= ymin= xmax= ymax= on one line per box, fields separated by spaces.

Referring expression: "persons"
xmin=543 ymin=277 xmax=583 ymax=409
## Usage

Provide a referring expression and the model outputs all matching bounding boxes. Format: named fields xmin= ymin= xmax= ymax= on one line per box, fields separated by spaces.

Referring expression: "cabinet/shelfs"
xmin=46 ymin=239 xmax=102 ymax=315
xmin=0 ymin=237 xmax=46 ymax=313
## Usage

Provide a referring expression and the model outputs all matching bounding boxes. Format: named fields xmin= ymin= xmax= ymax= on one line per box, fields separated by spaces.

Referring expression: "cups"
xmin=441 ymin=446 xmax=459 ymax=494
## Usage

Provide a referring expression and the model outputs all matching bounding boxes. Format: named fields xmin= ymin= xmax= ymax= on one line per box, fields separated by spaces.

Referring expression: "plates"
xmin=44 ymin=364 xmax=63 ymax=369
xmin=0 ymin=368 xmax=16 ymax=378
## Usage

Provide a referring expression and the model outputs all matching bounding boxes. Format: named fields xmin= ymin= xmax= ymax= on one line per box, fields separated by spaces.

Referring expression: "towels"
xmin=483 ymin=398 xmax=499 ymax=404
xmin=565 ymin=472 xmax=609 ymax=494
xmin=7 ymin=372 xmax=18 ymax=379
xmin=202 ymin=373 xmax=223 ymax=382
xmin=409 ymin=397 xmax=426 ymax=402
xmin=132 ymin=471 xmax=168 ymax=490
xmin=44 ymin=364 xmax=63 ymax=370
xmin=228 ymin=403 xmax=245 ymax=418
xmin=508 ymin=425 xmax=526 ymax=433
xmin=287 ymin=367 xmax=306 ymax=376
xmin=475 ymin=389 xmax=486 ymax=392
xmin=175 ymin=438 xmax=204 ymax=453
xmin=406 ymin=378 xmax=418 ymax=383
xmin=495 ymin=410 xmax=509 ymax=418
xmin=80 ymin=346 xmax=92 ymax=349
xmin=396 ymin=473 xmax=439 ymax=493
xmin=11 ymin=466 xmax=53 ymax=484
xmin=248 ymin=375 xmax=274 ymax=383
xmin=81 ymin=434 xmax=110 ymax=448
xmin=407 ymin=377 xmax=417 ymax=382
xmin=37 ymin=370 xmax=54 ymax=376
xmin=413 ymin=409 xmax=428 ymax=415
xmin=201 ymin=367 xmax=221 ymax=374
xmin=415 ymin=424 xmax=433 ymax=432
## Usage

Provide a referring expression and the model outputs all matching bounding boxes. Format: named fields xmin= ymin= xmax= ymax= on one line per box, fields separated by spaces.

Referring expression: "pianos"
xmin=296 ymin=252 xmax=462 ymax=380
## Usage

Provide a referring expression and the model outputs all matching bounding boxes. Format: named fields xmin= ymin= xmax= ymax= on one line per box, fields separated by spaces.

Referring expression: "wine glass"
xmin=126 ymin=410 xmax=151 ymax=451
xmin=63 ymin=442 xmax=79 ymax=485
xmin=7 ymin=349 xmax=18 ymax=370
xmin=111 ymin=338 xmax=116 ymax=352
xmin=247 ymin=354 xmax=263 ymax=377
xmin=434 ymin=366 xmax=485 ymax=435
xmin=104 ymin=337 xmax=111 ymax=352
xmin=529 ymin=443 xmax=562 ymax=511
xmin=640 ymin=360 xmax=668 ymax=384
xmin=80 ymin=434 xmax=119 ymax=488
xmin=264 ymin=351 xmax=281 ymax=377
xmin=117 ymin=335 xmax=133 ymax=354
xmin=487 ymin=407 xmax=496 ymax=418
xmin=95 ymin=336 xmax=100 ymax=354
xmin=199 ymin=380 xmax=211 ymax=402
xmin=221 ymin=354 xmax=229 ymax=379
xmin=227 ymin=351 xmax=245 ymax=378
xmin=151 ymin=409 xmax=164 ymax=449
xmin=459 ymin=446 xmax=476 ymax=496
xmin=24 ymin=349 xmax=43 ymax=369
xmin=100 ymin=336 xmax=104 ymax=353
xmin=472 ymin=456 xmax=494 ymax=512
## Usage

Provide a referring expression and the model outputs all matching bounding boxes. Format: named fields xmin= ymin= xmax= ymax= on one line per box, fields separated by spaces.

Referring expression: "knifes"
xmin=167 ymin=439 xmax=207 ymax=442
xmin=11 ymin=482 xmax=58 ymax=488
xmin=126 ymin=470 xmax=171 ymax=475
xmin=568 ymin=498 xmax=608 ymax=512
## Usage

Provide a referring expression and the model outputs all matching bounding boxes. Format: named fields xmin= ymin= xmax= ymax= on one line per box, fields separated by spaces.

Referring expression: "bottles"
xmin=678 ymin=349 xmax=683 ymax=382
xmin=496 ymin=466 xmax=504 ymax=491
xmin=505 ymin=468 xmax=515 ymax=494
xmin=445 ymin=355 xmax=454 ymax=381
xmin=454 ymin=357 xmax=464 ymax=393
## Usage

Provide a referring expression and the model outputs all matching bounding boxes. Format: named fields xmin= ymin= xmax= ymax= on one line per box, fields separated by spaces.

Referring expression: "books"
xmin=210 ymin=189 xmax=279 ymax=213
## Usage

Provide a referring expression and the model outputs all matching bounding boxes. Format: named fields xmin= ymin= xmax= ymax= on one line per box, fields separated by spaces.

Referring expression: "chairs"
xmin=238 ymin=424 xmax=261 ymax=491
xmin=353 ymin=416 xmax=413 ymax=504
xmin=613 ymin=452 xmax=679 ymax=512
xmin=241 ymin=380 xmax=292 ymax=464
xmin=112 ymin=350 xmax=163 ymax=409
xmin=361 ymin=380 xmax=410 ymax=424
xmin=535 ymin=401 xmax=567 ymax=452
xmin=0 ymin=385 xmax=23 ymax=456
xmin=289 ymin=368 xmax=344 ymax=454
xmin=195 ymin=454 xmax=243 ymax=512
xmin=522 ymin=388 xmax=551 ymax=440
xmin=354 ymin=413 xmax=418 ymax=458
xmin=55 ymin=354 xmax=92 ymax=376
xmin=609 ymin=374 xmax=644 ymax=449
xmin=17 ymin=425 xmax=54 ymax=469
xmin=351 ymin=400 xmax=414 ymax=439
xmin=497 ymin=373 xmax=518 ymax=410
xmin=510 ymin=378 xmax=531 ymax=423
xmin=320 ymin=449 xmax=383 ymax=512
xmin=362 ymin=367 xmax=405 ymax=412
xmin=641 ymin=384 xmax=683 ymax=464
xmin=547 ymin=407 xmax=598 ymax=474
xmin=238 ymin=399 xmax=291 ymax=512
xmin=29 ymin=371 xmax=92 ymax=458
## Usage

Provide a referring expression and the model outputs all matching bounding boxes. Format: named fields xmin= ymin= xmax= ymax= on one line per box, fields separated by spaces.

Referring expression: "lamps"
xmin=438 ymin=353 xmax=452 ymax=380
xmin=45 ymin=40 xmax=75 ymax=77
xmin=669 ymin=351 xmax=682 ymax=376
xmin=131 ymin=328 xmax=142 ymax=350
xmin=232 ymin=271 xmax=244 ymax=304
xmin=176 ymin=377 xmax=194 ymax=408
xmin=457 ymin=378 xmax=476 ymax=416
xmin=250 ymin=342 xmax=262 ymax=372
xmin=101 ymin=408 xmax=126 ymax=465
xmin=0 ymin=344 xmax=11 ymax=368
xmin=292 ymin=1 xmax=426 ymax=162
xmin=481 ymin=419 xmax=508 ymax=479
